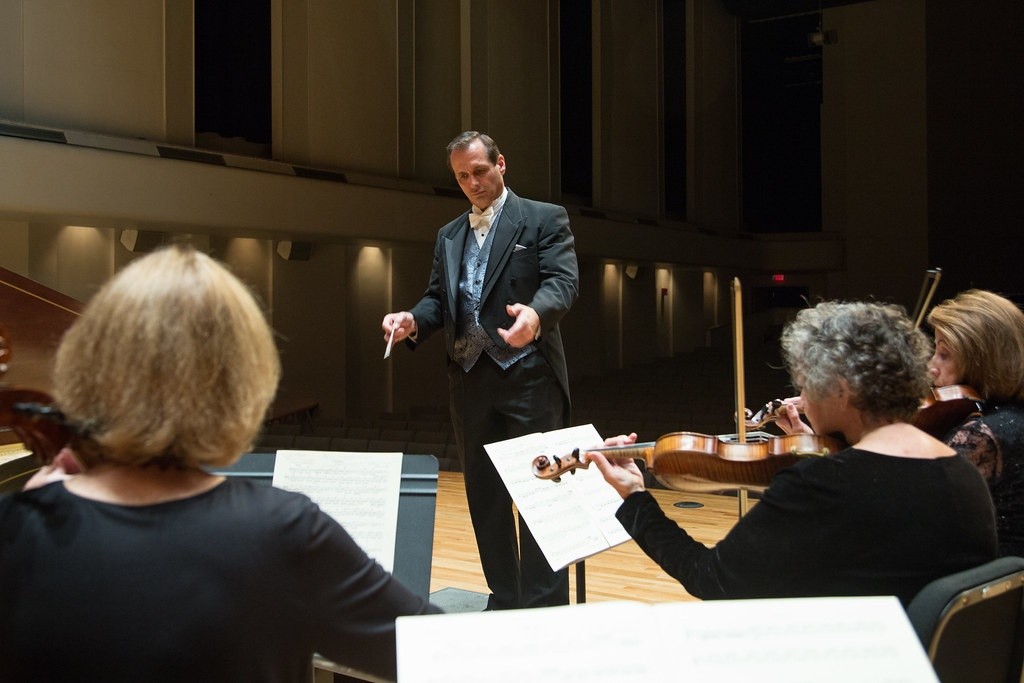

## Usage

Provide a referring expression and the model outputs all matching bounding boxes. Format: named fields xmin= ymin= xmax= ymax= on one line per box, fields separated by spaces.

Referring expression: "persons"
xmin=585 ymin=301 xmax=998 ymax=610
xmin=382 ymin=131 xmax=578 ymax=611
xmin=0 ymin=243 xmax=441 ymax=683
xmin=773 ymin=288 xmax=1024 ymax=555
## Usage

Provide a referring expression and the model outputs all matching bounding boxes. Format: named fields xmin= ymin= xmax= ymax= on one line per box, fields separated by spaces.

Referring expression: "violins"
xmin=530 ymin=427 xmax=846 ymax=498
xmin=732 ymin=381 xmax=989 ymax=438
xmin=2 ymin=335 xmax=96 ymax=469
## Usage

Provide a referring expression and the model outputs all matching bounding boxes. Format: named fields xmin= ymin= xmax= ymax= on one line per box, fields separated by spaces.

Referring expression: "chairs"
xmin=907 ymin=556 xmax=1024 ymax=683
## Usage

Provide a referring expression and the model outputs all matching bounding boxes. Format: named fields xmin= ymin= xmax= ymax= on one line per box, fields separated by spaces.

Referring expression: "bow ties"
xmin=469 ymin=206 xmax=494 ymax=228
